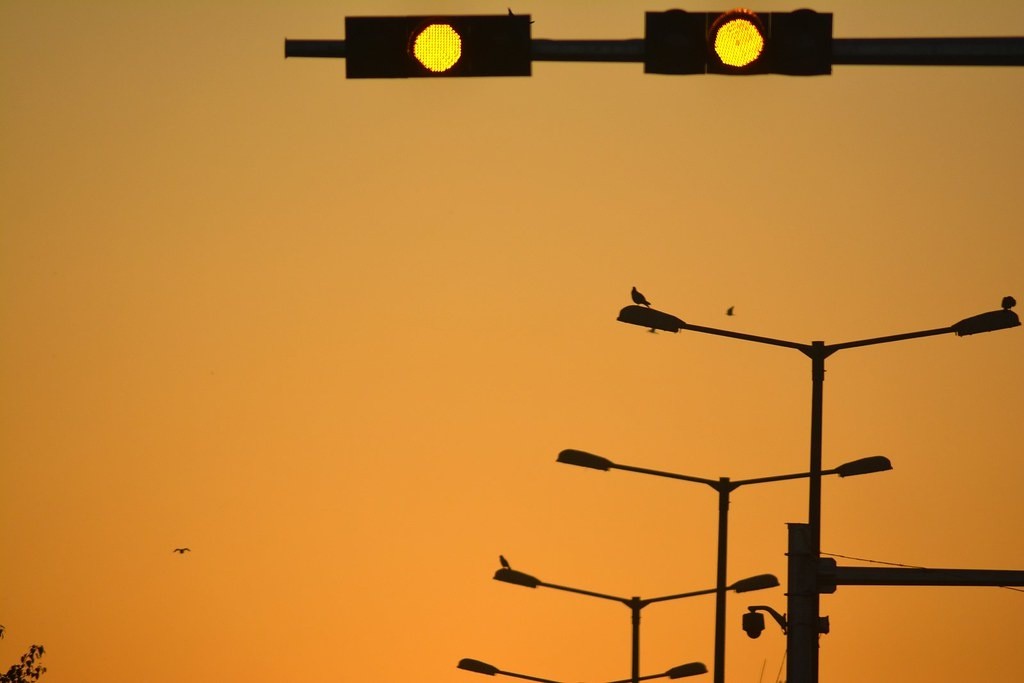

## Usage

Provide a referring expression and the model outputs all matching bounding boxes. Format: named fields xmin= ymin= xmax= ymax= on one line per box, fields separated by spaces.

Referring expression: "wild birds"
xmin=1001 ymin=296 xmax=1016 ymax=310
xmin=173 ymin=548 xmax=190 ymax=554
xmin=726 ymin=306 xmax=735 ymax=316
xmin=631 ymin=286 xmax=651 ymax=308
xmin=499 ymin=555 xmax=511 ymax=570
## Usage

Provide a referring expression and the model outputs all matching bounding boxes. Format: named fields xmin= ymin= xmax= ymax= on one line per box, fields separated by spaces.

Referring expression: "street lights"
xmin=496 ymin=554 xmax=780 ymax=683
xmin=555 ymin=445 xmax=892 ymax=683
xmin=618 ymin=287 xmax=1021 ymax=682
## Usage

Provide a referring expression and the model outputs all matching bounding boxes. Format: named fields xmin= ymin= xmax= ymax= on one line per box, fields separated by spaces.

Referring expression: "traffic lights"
xmin=644 ymin=8 xmax=834 ymax=75
xmin=343 ymin=13 xmax=533 ymax=78
